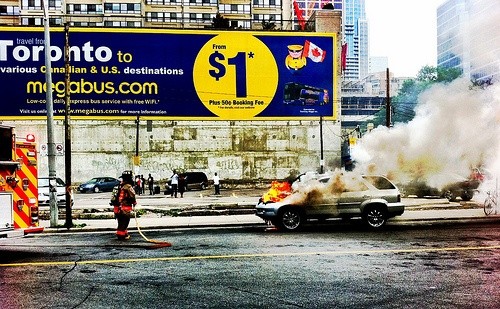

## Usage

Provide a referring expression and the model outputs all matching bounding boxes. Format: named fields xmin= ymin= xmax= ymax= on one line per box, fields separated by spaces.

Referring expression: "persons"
xmin=134 ymin=168 xmax=225 ymax=197
xmin=109 ymin=169 xmax=137 ymax=240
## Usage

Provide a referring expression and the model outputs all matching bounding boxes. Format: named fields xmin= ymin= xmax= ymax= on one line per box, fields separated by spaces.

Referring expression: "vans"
xmin=165 ymin=172 xmax=209 ymax=192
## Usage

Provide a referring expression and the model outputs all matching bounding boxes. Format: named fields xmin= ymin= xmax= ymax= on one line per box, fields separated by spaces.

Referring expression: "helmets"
xmin=119 ymin=170 xmax=135 ymax=178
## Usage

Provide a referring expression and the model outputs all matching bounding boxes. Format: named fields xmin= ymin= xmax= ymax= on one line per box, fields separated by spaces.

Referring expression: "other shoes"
xmin=116 ymin=234 xmax=131 ymax=239
xmin=180 ymin=196 xmax=183 ymax=198
xmin=174 ymin=195 xmax=177 ymax=198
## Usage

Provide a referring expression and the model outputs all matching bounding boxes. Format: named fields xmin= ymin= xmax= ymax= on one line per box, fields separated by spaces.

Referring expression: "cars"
xmin=254 ymin=174 xmax=405 ymax=232
xmin=394 ymin=172 xmax=480 ymax=203
xmin=39 ymin=176 xmax=74 ymax=208
xmin=77 ymin=177 xmax=121 ymax=193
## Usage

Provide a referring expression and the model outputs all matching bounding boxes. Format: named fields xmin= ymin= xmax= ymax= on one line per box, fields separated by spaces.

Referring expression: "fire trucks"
xmin=0 ymin=125 xmax=45 ymax=238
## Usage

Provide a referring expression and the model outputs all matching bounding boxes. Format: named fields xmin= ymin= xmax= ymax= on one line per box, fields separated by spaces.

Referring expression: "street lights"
xmin=61 ymin=22 xmax=76 ymax=228
